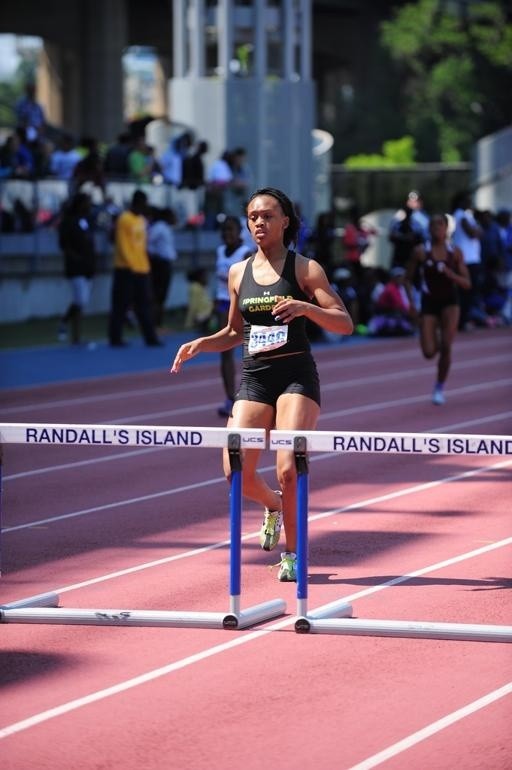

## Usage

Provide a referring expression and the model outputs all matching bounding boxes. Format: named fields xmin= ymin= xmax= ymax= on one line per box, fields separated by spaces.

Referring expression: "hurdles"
xmin=0 ymin=422 xmax=288 ymax=630
xmin=270 ymin=430 xmax=512 ymax=643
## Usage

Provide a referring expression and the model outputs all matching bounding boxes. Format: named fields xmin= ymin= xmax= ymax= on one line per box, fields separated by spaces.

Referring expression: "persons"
xmin=207 ymin=215 xmax=253 ymax=419
xmin=290 ymin=191 xmax=512 ymax=341
xmin=169 ymin=186 xmax=357 ymax=582
xmin=402 ymin=211 xmax=474 ymax=405
xmin=1 ymin=82 xmax=250 ymax=348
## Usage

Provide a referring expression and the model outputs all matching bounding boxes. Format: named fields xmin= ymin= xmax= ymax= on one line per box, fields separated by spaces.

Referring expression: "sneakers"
xmin=432 ymin=391 xmax=443 ymax=405
xmin=269 ymin=552 xmax=297 ymax=581
xmin=260 ymin=490 xmax=283 ymax=551
xmin=56 ymin=322 xmax=164 ymax=352
xmin=218 ymin=399 xmax=233 ymax=417
